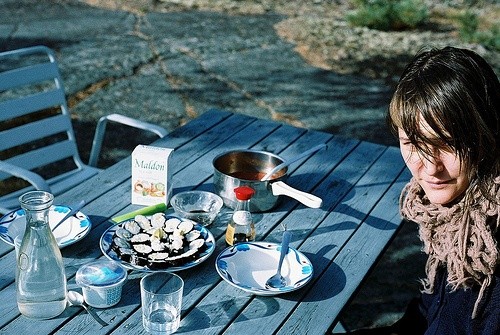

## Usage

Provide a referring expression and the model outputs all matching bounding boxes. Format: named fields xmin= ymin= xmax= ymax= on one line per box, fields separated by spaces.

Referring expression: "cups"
xmin=76 ymin=261 xmax=128 ymax=308
xmin=140 ymin=272 xmax=184 ymax=335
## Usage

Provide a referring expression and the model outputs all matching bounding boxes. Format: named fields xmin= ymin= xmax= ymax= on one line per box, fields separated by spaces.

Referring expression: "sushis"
xmin=111 ymin=212 xmax=207 ymax=270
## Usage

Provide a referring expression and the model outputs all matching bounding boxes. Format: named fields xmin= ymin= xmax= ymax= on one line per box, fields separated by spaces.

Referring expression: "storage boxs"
xmin=132 ymin=145 xmax=174 ymax=208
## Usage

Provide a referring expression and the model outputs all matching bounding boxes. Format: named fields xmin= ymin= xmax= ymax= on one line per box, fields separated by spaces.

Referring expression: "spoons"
xmin=66 ymin=290 xmax=108 ymax=327
xmin=266 ymin=230 xmax=293 ymax=289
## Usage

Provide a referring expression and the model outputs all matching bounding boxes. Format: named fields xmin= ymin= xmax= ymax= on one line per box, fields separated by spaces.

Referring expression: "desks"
xmin=0 ymin=108 xmax=413 ymax=335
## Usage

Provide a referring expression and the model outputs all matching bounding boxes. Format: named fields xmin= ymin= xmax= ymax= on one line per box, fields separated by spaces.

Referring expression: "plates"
xmin=99 ymin=216 xmax=215 ymax=272
xmin=215 ymin=240 xmax=314 ymax=296
xmin=0 ymin=204 xmax=92 ymax=249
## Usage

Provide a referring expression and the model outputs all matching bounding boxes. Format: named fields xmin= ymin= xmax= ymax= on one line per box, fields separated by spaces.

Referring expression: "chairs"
xmin=0 ymin=46 xmax=168 ymax=216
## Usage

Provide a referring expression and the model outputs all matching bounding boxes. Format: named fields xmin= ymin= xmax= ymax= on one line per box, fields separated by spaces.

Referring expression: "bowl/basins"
xmin=171 ymin=191 xmax=223 ymax=227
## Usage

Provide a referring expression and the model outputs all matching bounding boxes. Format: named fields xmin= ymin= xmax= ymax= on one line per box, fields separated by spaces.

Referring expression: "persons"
xmin=350 ymin=47 xmax=500 ymax=335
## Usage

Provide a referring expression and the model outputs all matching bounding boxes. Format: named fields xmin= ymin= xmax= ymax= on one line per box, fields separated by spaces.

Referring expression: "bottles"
xmin=225 ymin=186 xmax=256 ymax=248
xmin=15 ymin=190 xmax=67 ymax=319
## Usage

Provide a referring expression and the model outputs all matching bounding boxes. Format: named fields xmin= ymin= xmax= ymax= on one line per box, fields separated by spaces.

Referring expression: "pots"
xmin=212 ymin=149 xmax=324 ymax=212
